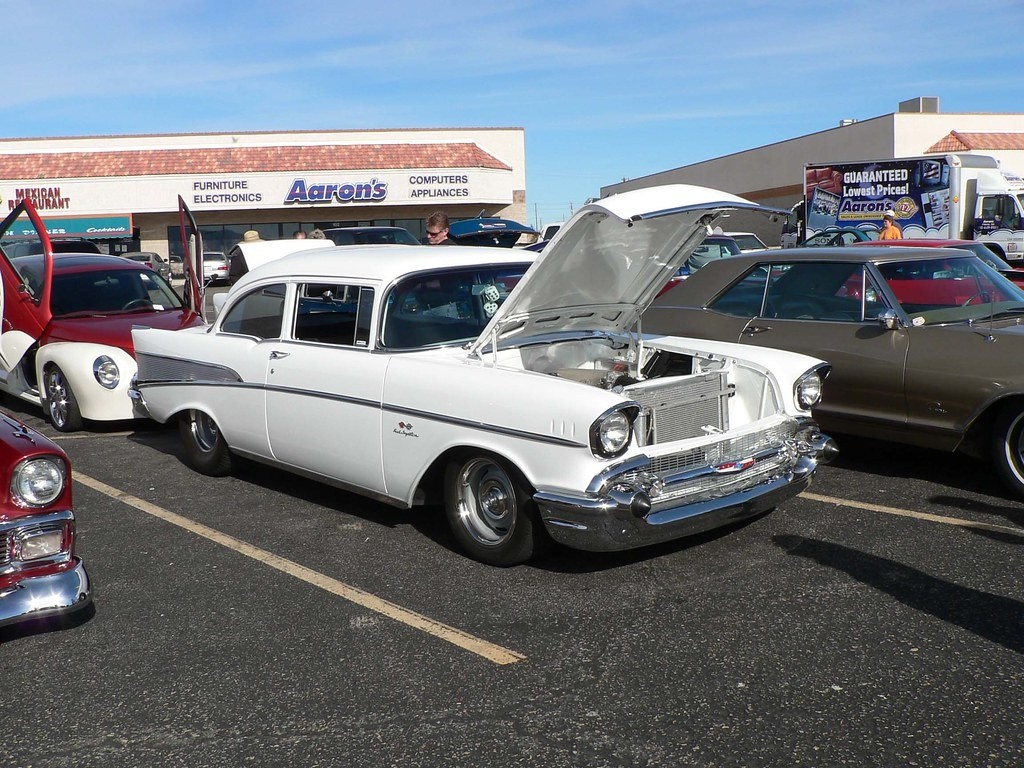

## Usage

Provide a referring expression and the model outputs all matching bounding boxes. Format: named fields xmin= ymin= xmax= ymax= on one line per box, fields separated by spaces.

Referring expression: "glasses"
xmin=426 ymin=227 xmax=449 ymax=236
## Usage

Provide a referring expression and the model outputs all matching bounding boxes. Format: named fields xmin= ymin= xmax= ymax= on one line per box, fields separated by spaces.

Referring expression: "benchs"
xmin=224 ymin=311 xmax=356 ymax=345
xmin=710 ymin=294 xmax=847 ymax=319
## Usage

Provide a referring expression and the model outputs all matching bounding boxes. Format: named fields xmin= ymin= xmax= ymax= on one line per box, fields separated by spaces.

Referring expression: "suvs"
xmin=2 ymin=236 xmax=102 ymax=261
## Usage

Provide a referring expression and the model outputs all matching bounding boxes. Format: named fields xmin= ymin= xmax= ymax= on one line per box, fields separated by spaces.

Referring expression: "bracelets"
xmin=420 ymin=282 xmax=426 ymax=288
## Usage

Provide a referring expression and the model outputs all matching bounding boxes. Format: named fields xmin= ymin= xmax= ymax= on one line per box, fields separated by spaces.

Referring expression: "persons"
xmin=415 ymin=210 xmax=464 ymax=319
xmin=293 ymin=231 xmax=306 ymax=239
xmin=229 ymin=230 xmax=263 ymax=282
xmin=308 ymin=228 xmax=326 ymax=239
xmin=878 ymin=210 xmax=903 ymax=240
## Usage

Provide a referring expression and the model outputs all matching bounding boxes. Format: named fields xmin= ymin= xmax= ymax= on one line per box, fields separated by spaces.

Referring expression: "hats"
xmin=238 ymin=231 xmax=265 ymax=243
xmin=884 ymin=210 xmax=894 ymax=216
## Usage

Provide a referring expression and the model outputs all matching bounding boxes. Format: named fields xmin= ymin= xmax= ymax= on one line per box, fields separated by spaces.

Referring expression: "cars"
xmin=119 ymin=252 xmax=174 ymax=287
xmin=129 ymin=181 xmax=840 ymax=566
xmin=316 ymin=223 xmax=426 ymax=249
xmin=651 ymin=237 xmax=1024 ymax=312
xmin=632 ymin=243 xmax=1024 ymax=498
xmin=203 ymin=250 xmax=232 ymax=285
xmin=0 ymin=398 xmax=94 ymax=629
xmin=405 ymin=215 xmax=782 ymax=330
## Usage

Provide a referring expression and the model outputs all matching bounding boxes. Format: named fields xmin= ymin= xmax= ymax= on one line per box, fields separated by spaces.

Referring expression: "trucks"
xmin=770 ymin=151 xmax=1024 ymax=266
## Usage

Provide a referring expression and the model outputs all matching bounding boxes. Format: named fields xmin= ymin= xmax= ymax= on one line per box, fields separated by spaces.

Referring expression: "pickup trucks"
xmin=170 ymin=255 xmax=186 ymax=277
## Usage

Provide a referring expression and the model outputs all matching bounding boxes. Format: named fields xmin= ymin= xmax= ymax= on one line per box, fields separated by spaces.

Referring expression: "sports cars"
xmin=0 ymin=194 xmax=218 ymax=432
